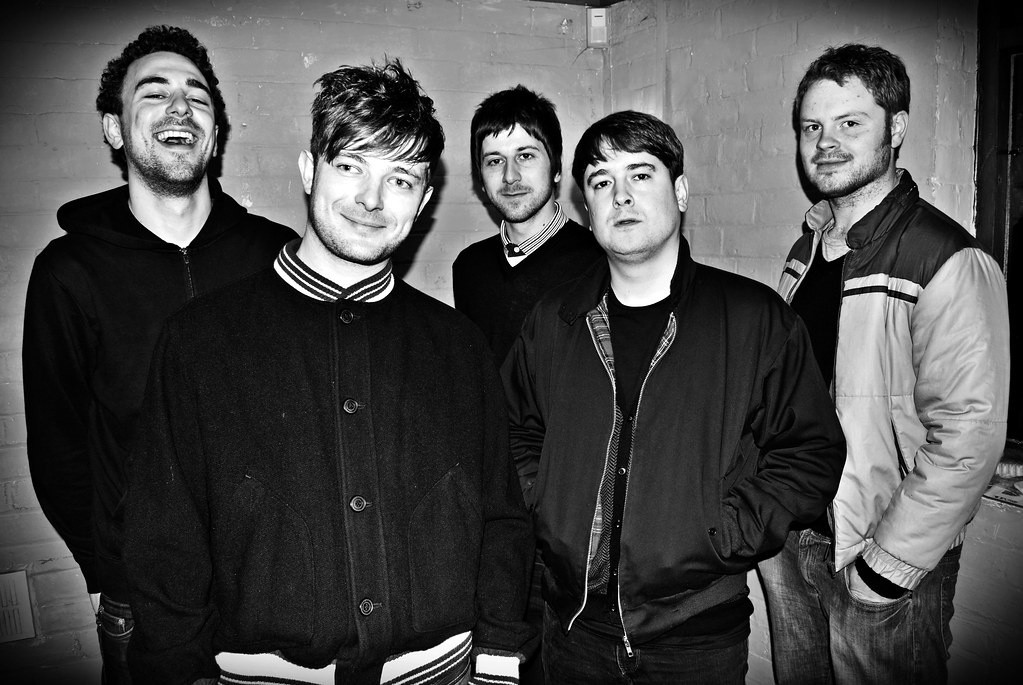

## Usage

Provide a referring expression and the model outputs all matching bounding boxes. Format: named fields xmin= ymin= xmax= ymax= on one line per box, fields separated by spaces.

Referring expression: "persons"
xmin=501 ymin=111 xmax=846 ymax=685
xmin=451 ymin=86 xmax=597 ymax=352
xmin=25 ymin=28 xmax=301 ymax=685
xmin=757 ymin=43 xmax=1009 ymax=685
xmin=134 ymin=65 xmax=535 ymax=685
